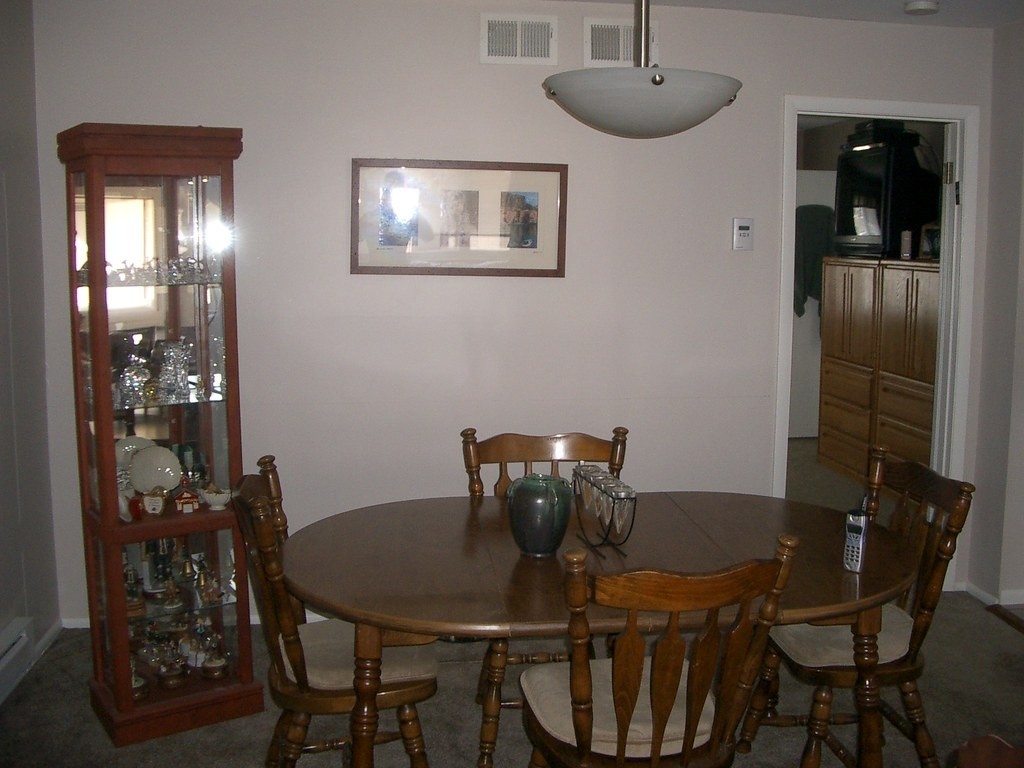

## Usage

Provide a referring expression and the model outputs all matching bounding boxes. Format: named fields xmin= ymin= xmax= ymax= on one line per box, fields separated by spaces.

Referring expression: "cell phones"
xmin=844 ymin=494 xmax=871 ymax=573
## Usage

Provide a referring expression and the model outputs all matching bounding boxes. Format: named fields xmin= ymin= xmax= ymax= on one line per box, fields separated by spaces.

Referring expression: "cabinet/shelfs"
xmin=817 ymin=256 xmax=939 ymax=504
xmin=55 ymin=122 xmax=267 ymax=749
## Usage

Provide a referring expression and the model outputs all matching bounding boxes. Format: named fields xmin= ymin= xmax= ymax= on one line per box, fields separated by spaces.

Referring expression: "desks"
xmin=279 ymin=490 xmax=919 ymax=768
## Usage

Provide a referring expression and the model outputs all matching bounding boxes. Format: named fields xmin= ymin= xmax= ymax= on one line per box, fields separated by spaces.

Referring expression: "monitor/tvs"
xmin=833 ymin=142 xmax=933 ymax=261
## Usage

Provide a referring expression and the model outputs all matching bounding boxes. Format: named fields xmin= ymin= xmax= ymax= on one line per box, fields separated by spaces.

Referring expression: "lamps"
xmin=542 ymin=0 xmax=743 ymax=138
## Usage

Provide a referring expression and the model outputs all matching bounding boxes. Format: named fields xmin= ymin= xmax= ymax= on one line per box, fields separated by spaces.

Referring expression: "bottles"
xmin=505 ymin=472 xmax=573 ymax=559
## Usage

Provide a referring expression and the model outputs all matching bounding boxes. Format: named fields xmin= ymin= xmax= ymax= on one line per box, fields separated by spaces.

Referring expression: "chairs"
xmin=228 ymin=454 xmax=439 ymax=768
xmin=513 ymin=530 xmax=799 ymax=768
xmin=459 ymin=427 xmax=628 ymax=768
xmin=735 ymin=443 xmax=975 ymax=768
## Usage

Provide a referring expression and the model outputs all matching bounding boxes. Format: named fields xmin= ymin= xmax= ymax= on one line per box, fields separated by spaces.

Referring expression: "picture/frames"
xmin=351 ymin=157 xmax=568 ymax=277
